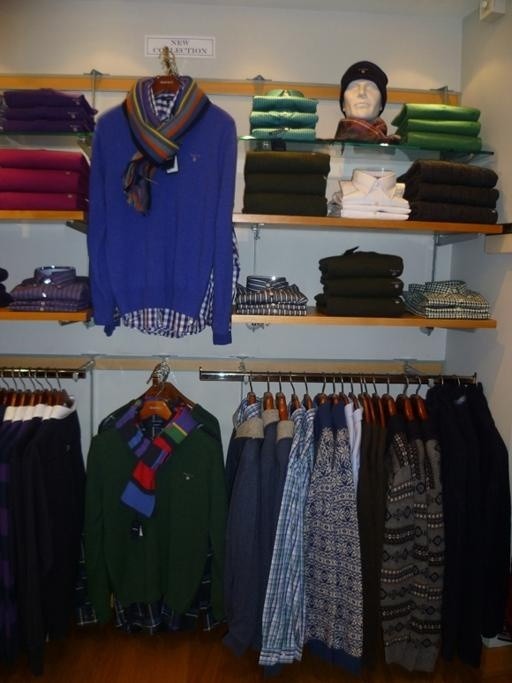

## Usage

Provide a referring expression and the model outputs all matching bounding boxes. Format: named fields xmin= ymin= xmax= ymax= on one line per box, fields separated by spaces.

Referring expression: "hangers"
xmin=1 ymin=365 xmax=73 ymax=410
xmin=137 ymin=362 xmax=196 ymax=425
xmin=241 ymin=367 xmax=481 ymax=424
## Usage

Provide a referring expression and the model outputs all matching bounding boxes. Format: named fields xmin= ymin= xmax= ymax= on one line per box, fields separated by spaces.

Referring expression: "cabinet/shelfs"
xmin=2 ymin=209 xmax=88 ymax=232
xmin=231 ymin=307 xmax=498 ymax=336
xmin=232 ymin=213 xmax=503 ymax=244
xmin=2 ymin=306 xmax=92 ymax=326
xmin=2 ymin=131 xmax=91 ymax=147
xmin=238 ymin=136 xmax=493 ymax=165
